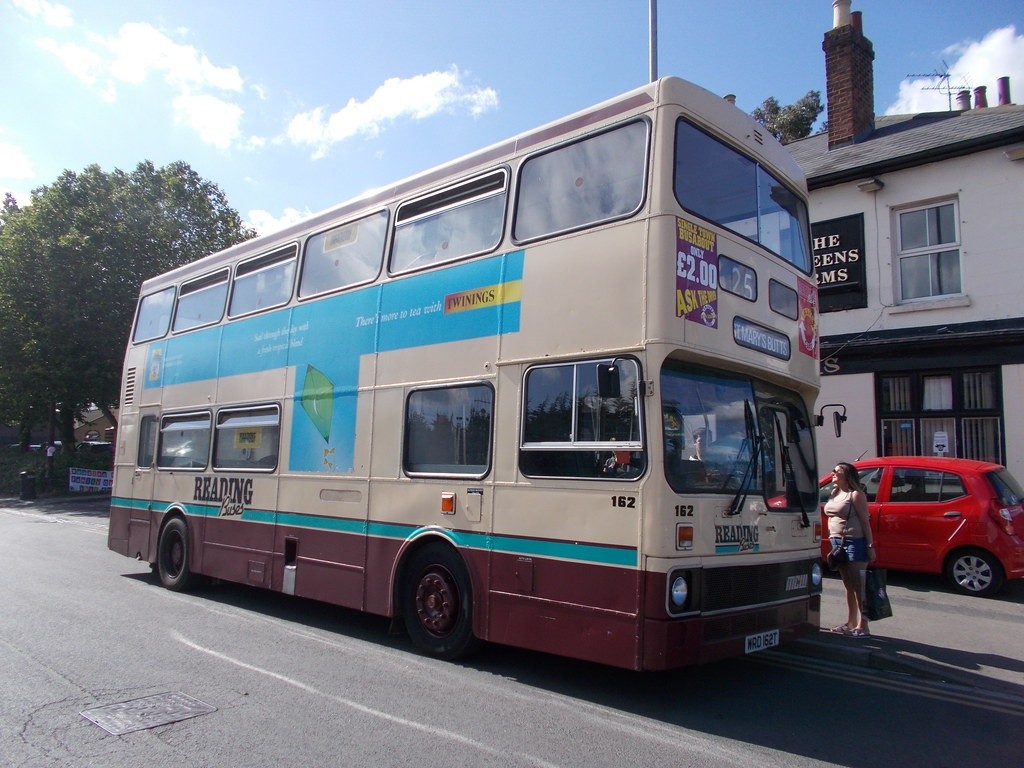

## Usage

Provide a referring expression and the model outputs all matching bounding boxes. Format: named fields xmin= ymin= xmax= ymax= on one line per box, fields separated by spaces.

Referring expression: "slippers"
xmin=828 ymin=624 xmax=851 ymax=633
xmin=843 ymin=628 xmax=871 ymax=638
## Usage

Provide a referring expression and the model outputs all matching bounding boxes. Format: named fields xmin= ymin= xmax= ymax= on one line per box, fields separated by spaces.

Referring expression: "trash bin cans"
xmin=19 ymin=471 xmax=37 ymax=500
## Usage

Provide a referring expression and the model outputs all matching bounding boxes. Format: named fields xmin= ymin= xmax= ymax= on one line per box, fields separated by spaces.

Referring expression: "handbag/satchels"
xmin=826 ymin=545 xmax=848 ymax=571
xmin=863 ymin=559 xmax=893 ymax=621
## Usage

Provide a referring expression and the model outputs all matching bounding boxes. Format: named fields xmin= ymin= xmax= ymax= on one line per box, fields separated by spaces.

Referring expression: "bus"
xmin=108 ymin=74 xmax=849 ymax=672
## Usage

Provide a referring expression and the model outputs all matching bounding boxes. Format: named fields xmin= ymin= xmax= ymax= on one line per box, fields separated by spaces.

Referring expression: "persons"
xmin=602 ymin=436 xmax=643 ymax=476
xmin=690 ymin=427 xmax=712 ymax=462
xmin=702 ymin=418 xmax=772 ymax=488
xmin=824 ymin=462 xmax=875 ymax=639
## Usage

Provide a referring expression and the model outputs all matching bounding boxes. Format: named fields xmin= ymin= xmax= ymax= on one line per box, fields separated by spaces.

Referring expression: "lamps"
xmin=1002 ymin=146 xmax=1024 ymax=161
xmin=857 ymin=178 xmax=883 ymax=192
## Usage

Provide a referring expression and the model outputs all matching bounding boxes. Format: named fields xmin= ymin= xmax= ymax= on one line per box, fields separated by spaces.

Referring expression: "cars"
xmin=767 ymin=454 xmax=1024 ymax=597
xmin=41 ymin=440 xmax=112 ymax=458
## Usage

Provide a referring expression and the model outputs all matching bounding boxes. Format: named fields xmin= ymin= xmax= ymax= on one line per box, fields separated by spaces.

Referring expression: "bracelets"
xmin=867 ymin=543 xmax=876 ymax=548
